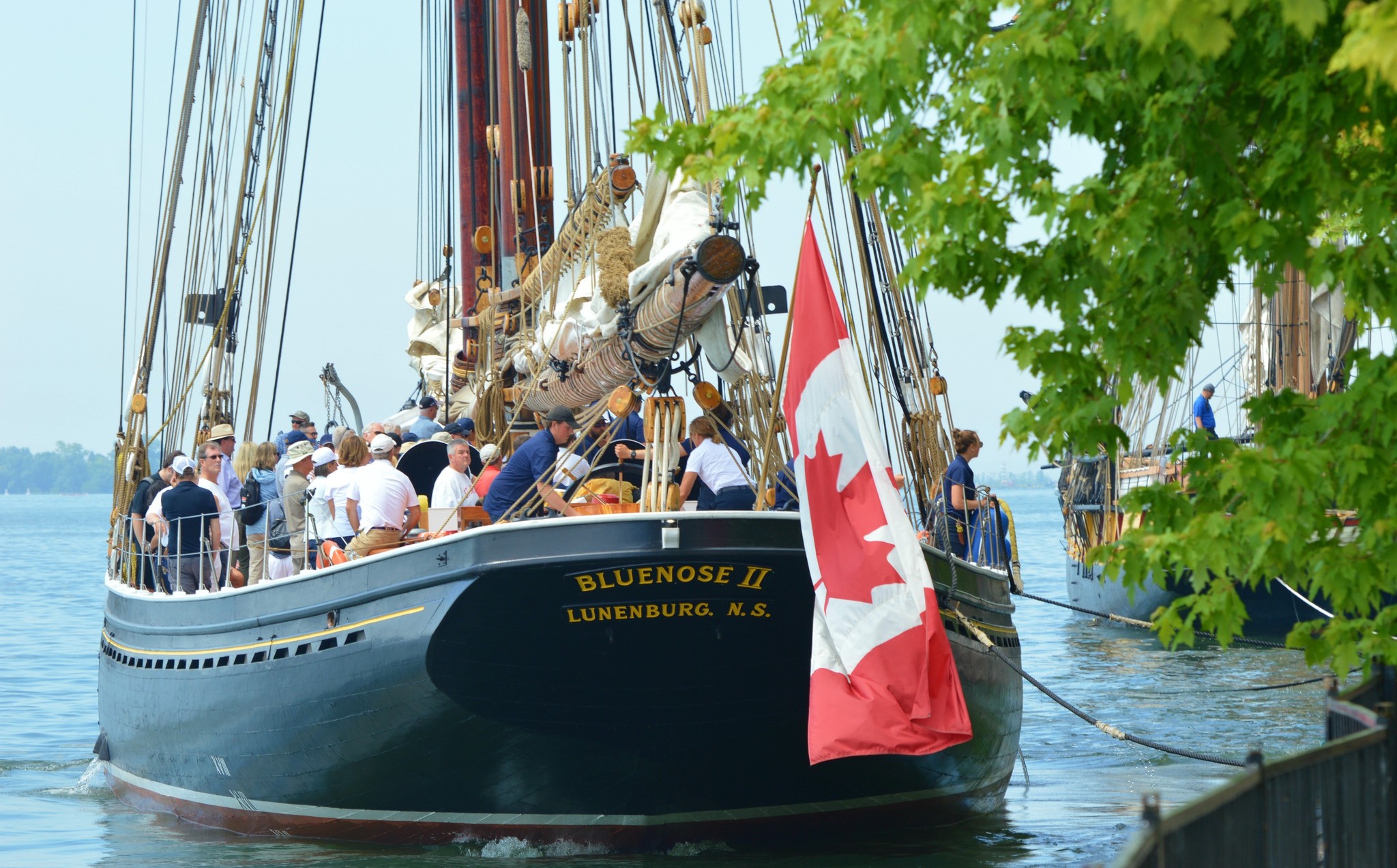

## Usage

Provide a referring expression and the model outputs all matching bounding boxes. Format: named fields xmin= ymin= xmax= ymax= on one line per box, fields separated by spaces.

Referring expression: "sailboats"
xmin=78 ymin=0 xmax=1026 ymax=855
xmin=1036 ymin=160 xmax=1389 ymax=641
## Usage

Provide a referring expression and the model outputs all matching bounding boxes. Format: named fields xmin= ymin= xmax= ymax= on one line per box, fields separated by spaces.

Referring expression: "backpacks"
xmin=240 ymin=472 xmax=266 ymax=525
xmin=269 ymin=499 xmax=292 ymax=555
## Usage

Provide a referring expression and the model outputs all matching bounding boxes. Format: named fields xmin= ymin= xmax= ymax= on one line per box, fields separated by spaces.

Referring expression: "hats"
xmin=419 ymin=395 xmax=443 ymax=409
xmin=584 ymin=408 xmax=608 ymax=421
xmin=547 ymin=405 xmax=583 ymax=429
xmin=370 ymin=434 xmax=397 ymax=454
xmin=399 ymin=442 xmax=417 ymax=453
xmin=284 ymin=440 xmax=315 ymax=466
xmin=1203 ymin=384 xmax=1215 ymax=393
xmin=311 ymin=447 xmax=339 ymax=467
xmin=285 ymin=430 xmax=316 ymax=445
xmin=388 ymin=432 xmax=402 ymax=446
xmin=456 ymin=417 xmax=475 ymax=430
xmin=283 ymin=467 xmax=294 ymax=479
xmin=206 ymin=424 xmax=238 ymax=442
xmin=319 ymin=434 xmax=334 ymax=446
xmin=634 ymin=391 xmax=645 ymax=402
xmin=289 ymin=411 xmax=310 ymax=422
xmin=443 ymin=422 xmax=471 ymax=435
xmin=430 ymin=431 xmax=453 ymax=444
xmin=168 ymin=455 xmax=195 ymax=474
xmin=480 ymin=443 xmax=501 ymax=463
xmin=400 ymin=432 xmax=419 ymax=444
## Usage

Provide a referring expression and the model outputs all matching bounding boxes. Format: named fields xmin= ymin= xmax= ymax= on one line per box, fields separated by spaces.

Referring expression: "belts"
xmin=716 ymin=485 xmax=751 ymax=495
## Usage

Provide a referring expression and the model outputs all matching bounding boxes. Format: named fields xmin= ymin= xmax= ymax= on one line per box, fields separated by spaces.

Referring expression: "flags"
xmin=783 ymin=222 xmax=972 ymax=767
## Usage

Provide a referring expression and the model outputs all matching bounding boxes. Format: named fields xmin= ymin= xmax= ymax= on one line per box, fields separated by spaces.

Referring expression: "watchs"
xmin=631 ymin=450 xmax=636 ymax=460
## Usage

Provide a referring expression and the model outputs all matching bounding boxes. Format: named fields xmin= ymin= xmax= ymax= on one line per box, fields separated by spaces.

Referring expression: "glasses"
xmin=594 ymin=422 xmax=607 ymax=428
xmin=339 ymin=427 xmax=350 ymax=443
xmin=979 ymin=442 xmax=983 ymax=447
xmin=274 ymin=451 xmax=280 ymax=457
xmin=304 ymin=432 xmax=318 ymax=437
xmin=205 ymin=454 xmax=224 ymax=460
xmin=437 ymin=405 xmax=440 ymax=410
xmin=367 ymin=431 xmax=387 ymax=435
xmin=292 ymin=420 xmax=303 ymax=425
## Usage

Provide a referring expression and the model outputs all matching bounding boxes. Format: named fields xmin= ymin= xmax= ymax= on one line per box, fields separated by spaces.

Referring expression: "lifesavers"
xmin=417 ymin=531 xmax=461 ymax=542
xmin=316 ymin=540 xmax=347 ymax=572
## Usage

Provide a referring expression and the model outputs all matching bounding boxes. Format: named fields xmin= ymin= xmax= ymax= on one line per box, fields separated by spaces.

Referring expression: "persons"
xmin=323 ymin=435 xmax=370 ymax=554
xmin=272 ymin=395 xmax=478 ymax=497
xmin=344 ymin=433 xmax=422 ymax=562
xmin=268 ymin=499 xmax=294 ymax=580
xmin=474 ymin=444 xmax=503 ymax=498
xmin=132 ymin=441 xmax=244 ymax=594
xmin=283 ymin=440 xmax=315 ymax=576
xmin=931 ymin=468 xmax=948 ymax=553
xmin=304 ymin=447 xmax=340 ymax=570
xmin=679 ymin=415 xmax=764 ymax=511
xmin=513 ymin=435 xmax=532 ymax=451
xmin=161 ymin=455 xmax=221 ymax=594
xmin=553 ymin=433 xmax=591 ymax=487
xmin=609 ymin=391 xmax=646 ymax=446
xmin=1194 ymin=383 xmax=1219 ymax=441
xmin=614 ymin=401 xmax=750 ymax=511
xmin=245 ymin=442 xmax=280 ymax=586
xmin=894 ymin=474 xmax=905 ymax=489
xmin=775 ymin=457 xmax=799 ymax=510
xmin=574 ymin=415 xmax=607 ymax=466
xmin=233 ymin=441 xmax=258 ymax=486
xmin=943 ymin=428 xmax=997 ymax=560
xmin=431 ymin=438 xmax=480 ymax=531
xmin=206 ymin=424 xmax=250 ymax=587
xmin=482 ymin=405 xmax=584 ymax=524
